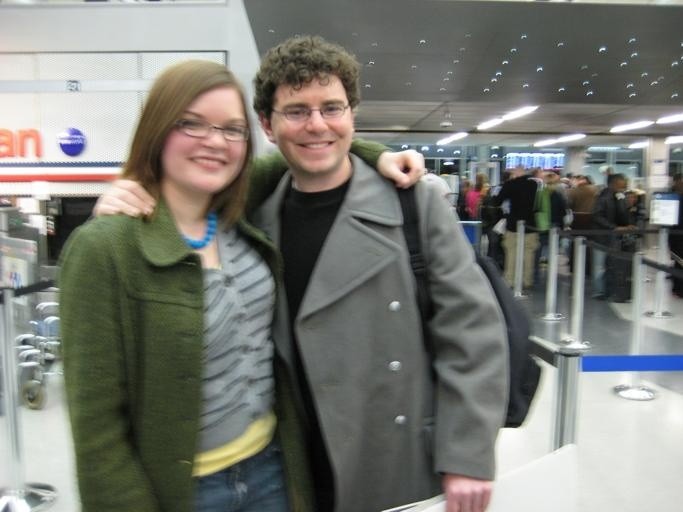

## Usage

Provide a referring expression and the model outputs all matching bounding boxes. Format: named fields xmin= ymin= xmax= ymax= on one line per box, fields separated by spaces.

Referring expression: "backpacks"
xmin=400 ymin=165 xmax=542 ymax=428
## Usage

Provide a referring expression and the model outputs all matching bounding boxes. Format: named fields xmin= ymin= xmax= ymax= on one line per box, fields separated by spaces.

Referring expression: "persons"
xmin=59 ymin=59 xmax=429 ymax=511
xmin=456 ymin=164 xmax=683 ymax=303
xmin=91 ymin=38 xmax=510 ymax=512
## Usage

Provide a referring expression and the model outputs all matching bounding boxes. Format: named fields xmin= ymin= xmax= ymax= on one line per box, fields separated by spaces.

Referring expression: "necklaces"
xmin=183 ymin=213 xmax=217 ymax=248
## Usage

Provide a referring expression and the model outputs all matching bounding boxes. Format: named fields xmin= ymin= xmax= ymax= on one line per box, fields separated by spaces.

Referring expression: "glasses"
xmin=173 ymin=118 xmax=251 ymax=142
xmin=272 ymin=101 xmax=351 ymax=122
